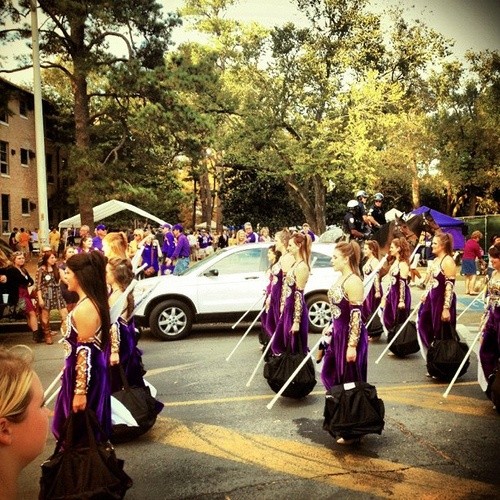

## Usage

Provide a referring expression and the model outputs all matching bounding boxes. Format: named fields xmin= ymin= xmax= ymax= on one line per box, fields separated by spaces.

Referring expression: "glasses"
xmin=101 ymin=228 xmax=107 ymax=231
xmin=376 ymin=200 xmax=381 ymax=203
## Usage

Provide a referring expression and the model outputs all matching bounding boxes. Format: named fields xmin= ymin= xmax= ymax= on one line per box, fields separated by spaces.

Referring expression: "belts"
xmin=178 ymin=255 xmax=188 ymax=258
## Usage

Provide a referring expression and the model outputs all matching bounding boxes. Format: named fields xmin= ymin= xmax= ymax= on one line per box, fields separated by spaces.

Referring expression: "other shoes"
xmin=32 ymin=330 xmax=45 ymax=343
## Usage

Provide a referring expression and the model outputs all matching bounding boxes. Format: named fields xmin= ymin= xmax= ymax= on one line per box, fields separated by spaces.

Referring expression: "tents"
xmin=408 ymin=206 xmax=465 ymax=250
xmin=59 ymin=201 xmax=170 ymax=228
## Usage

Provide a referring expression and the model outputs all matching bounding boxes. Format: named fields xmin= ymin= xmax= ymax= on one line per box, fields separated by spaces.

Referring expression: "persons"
xmin=367 ymin=193 xmax=386 ymax=235
xmin=480 ymin=242 xmax=500 ymax=416
xmin=383 ymin=237 xmax=411 ymax=356
xmin=129 ymin=221 xmax=271 ymax=280
xmin=300 ymin=222 xmax=315 ymax=242
xmin=53 ymin=246 xmax=80 ymax=313
xmin=0 ymin=239 xmax=20 ymax=318
xmin=344 ymin=199 xmax=366 ymax=239
xmin=318 ymin=239 xmax=369 ymax=445
xmin=9 ymin=224 xmax=107 ymax=267
xmin=51 ymin=250 xmax=113 ymax=450
xmin=357 ymin=190 xmax=372 ymax=238
xmin=417 ymin=233 xmax=457 ymax=379
xmin=0 ymin=343 xmax=50 ymax=500
xmin=105 ymin=257 xmax=165 ymax=416
xmin=259 ymin=227 xmax=318 ymax=399
xmin=9 ymin=250 xmax=46 ymax=344
xmin=462 ymin=230 xmax=484 ymax=295
xmin=362 ymin=240 xmax=384 ymax=342
xmin=487 ymin=234 xmax=500 ymax=280
xmin=36 ymin=249 xmax=68 ymax=344
xmin=101 ymin=232 xmax=129 ymax=259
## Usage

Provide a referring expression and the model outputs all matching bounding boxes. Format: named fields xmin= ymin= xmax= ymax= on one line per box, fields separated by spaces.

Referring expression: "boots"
xmin=40 ymin=319 xmax=52 ymax=344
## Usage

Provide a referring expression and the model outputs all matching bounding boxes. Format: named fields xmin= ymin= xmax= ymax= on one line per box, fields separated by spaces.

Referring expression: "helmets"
xmin=356 ymin=190 xmax=370 ymax=197
xmin=347 ymin=200 xmax=359 ymax=208
xmin=373 ymin=193 xmax=384 ymax=201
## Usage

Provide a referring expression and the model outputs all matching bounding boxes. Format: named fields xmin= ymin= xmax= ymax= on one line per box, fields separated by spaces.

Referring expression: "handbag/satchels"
xmin=322 ymin=361 xmax=386 ymax=437
xmin=426 ymin=320 xmax=471 ymax=380
xmin=364 ymin=297 xmax=384 ymax=336
xmin=258 ymin=328 xmax=267 ymax=344
xmin=108 ymin=362 xmax=157 ymax=443
xmin=387 ymin=308 xmax=420 ymax=356
xmin=486 ymin=360 xmax=500 ymax=411
xmin=39 ymin=408 xmax=133 ymax=500
xmin=262 ymin=330 xmax=317 ymax=399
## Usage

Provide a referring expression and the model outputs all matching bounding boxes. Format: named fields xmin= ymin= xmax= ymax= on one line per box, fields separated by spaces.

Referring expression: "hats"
xmin=97 ymin=224 xmax=106 ymax=229
xmin=171 ymin=224 xmax=183 ymax=231
xmin=162 ymin=224 xmax=170 ymax=228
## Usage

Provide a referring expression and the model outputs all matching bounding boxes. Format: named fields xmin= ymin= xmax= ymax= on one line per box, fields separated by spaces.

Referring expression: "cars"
xmin=133 ymin=242 xmax=342 ymax=342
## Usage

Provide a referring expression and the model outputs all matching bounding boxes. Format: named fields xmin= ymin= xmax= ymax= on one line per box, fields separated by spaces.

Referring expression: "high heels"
xmin=336 ymin=435 xmax=361 ymax=445
